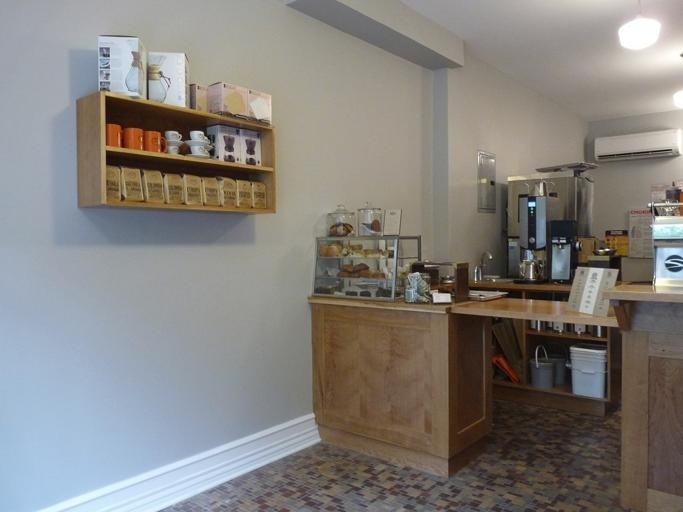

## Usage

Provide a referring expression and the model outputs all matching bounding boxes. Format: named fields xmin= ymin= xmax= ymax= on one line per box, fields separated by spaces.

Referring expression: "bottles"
xmin=328 ymin=201 xmax=384 ymax=237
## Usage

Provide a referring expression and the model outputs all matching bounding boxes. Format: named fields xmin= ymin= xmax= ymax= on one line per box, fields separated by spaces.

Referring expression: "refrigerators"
xmin=506 ymin=176 xmax=593 ymax=278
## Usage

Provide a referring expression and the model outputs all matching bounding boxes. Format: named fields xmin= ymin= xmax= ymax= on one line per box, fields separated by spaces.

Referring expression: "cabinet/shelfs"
xmin=76 ymin=90 xmax=280 ymax=215
xmin=311 ymin=234 xmax=422 ymax=302
xmin=467 ymin=280 xmax=634 ymax=417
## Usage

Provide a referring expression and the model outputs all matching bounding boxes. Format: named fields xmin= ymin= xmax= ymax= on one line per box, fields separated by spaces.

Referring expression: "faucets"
xmin=481 ymin=250 xmax=493 ymax=278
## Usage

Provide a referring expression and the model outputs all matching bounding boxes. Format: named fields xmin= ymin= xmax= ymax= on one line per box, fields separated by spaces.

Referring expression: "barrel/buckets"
xmin=567 ymin=340 xmax=608 ymax=399
xmin=551 ymin=354 xmax=566 ymax=386
xmin=531 ymin=346 xmax=554 ymax=389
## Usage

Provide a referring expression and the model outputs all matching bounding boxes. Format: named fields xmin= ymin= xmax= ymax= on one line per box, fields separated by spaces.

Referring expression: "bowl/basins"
xmin=594 ymin=247 xmax=610 ymax=255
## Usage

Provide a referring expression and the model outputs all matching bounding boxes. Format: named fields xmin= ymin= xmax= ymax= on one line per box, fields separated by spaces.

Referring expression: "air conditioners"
xmin=594 ymin=127 xmax=682 ymax=163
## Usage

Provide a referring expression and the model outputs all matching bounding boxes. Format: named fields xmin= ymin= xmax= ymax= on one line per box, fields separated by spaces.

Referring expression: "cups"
xmin=470 ymin=266 xmax=482 ymax=285
xmin=106 ymin=123 xmax=213 ymax=158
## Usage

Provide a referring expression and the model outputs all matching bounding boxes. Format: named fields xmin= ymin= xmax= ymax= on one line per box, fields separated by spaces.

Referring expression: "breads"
xmin=315 ymin=220 xmax=402 ymax=297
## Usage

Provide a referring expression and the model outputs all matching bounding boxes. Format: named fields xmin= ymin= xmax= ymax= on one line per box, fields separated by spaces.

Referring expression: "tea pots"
xmin=518 ymin=259 xmax=544 ymax=286
